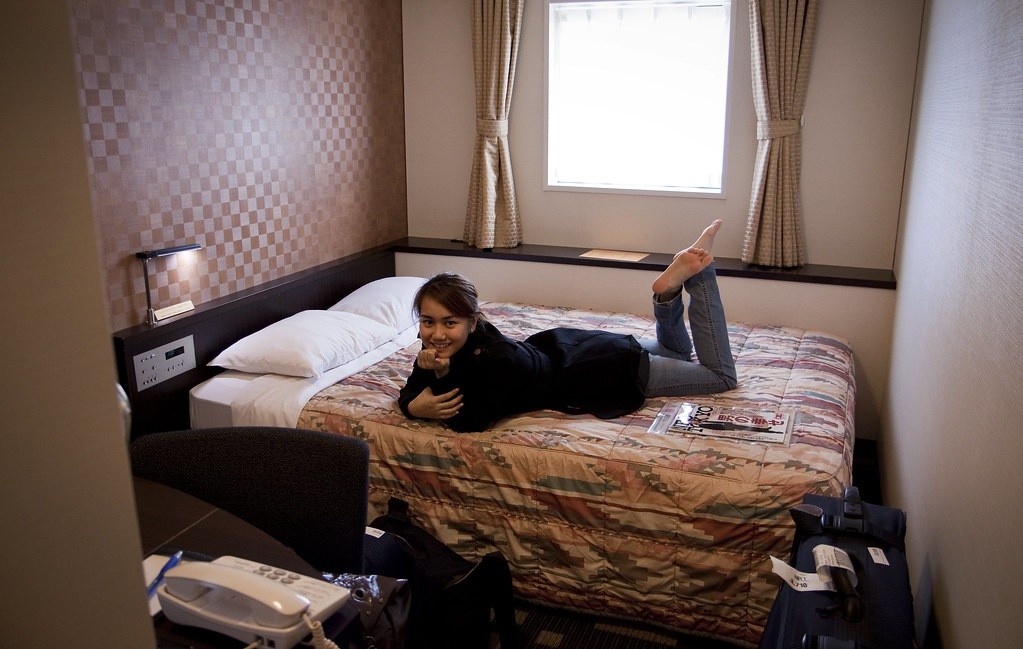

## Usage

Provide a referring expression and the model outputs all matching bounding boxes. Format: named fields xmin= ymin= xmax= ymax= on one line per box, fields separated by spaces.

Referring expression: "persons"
xmin=398 ymin=218 xmax=737 ymax=433
xmin=686 ymin=420 xmax=785 ymax=434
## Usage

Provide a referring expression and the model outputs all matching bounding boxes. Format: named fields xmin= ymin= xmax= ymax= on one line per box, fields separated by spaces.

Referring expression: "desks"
xmin=132 ymin=477 xmax=369 ymax=649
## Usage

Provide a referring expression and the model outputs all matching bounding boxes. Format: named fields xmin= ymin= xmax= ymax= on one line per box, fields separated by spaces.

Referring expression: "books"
xmin=667 ymin=401 xmax=790 ymax=444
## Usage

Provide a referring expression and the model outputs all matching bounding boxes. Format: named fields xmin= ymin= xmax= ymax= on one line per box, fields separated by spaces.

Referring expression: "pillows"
xmin=206 ymin=309 xmax=398 ymax=377
xmin=328 ymin=277 xmax=431 ymax=333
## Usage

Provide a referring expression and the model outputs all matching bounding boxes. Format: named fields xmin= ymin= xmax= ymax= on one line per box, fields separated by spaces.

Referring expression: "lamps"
xmin=135 ymin=243 xmax=201 ymax=327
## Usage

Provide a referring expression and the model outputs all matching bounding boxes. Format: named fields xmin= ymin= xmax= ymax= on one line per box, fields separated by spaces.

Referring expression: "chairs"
xmin=129 ymin=428 xmax=370 ymax=577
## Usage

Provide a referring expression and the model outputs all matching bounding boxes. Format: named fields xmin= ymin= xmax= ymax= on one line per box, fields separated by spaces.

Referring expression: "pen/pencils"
xmin=146 ymin=549 xmax=183 ymax=600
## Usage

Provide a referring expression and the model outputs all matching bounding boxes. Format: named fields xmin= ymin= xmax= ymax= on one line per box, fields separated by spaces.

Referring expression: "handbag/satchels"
xmin=322 ymin=572 xmax=411 ymax=649
xmin=364 ymin=497 xmax=521 ymax=649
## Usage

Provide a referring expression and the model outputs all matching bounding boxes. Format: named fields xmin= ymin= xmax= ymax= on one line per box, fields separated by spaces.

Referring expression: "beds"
xmin=111 ymin=250 xmax=855 ymax=649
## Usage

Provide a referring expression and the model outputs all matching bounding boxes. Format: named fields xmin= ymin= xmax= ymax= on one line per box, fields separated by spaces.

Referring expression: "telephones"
xmin=156 ymin=555 xmax=350 ymax=649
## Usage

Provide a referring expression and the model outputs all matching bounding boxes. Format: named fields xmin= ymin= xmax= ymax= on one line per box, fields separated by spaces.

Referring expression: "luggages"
xmin=758 ymin=486 xmax=914 ymax=649
